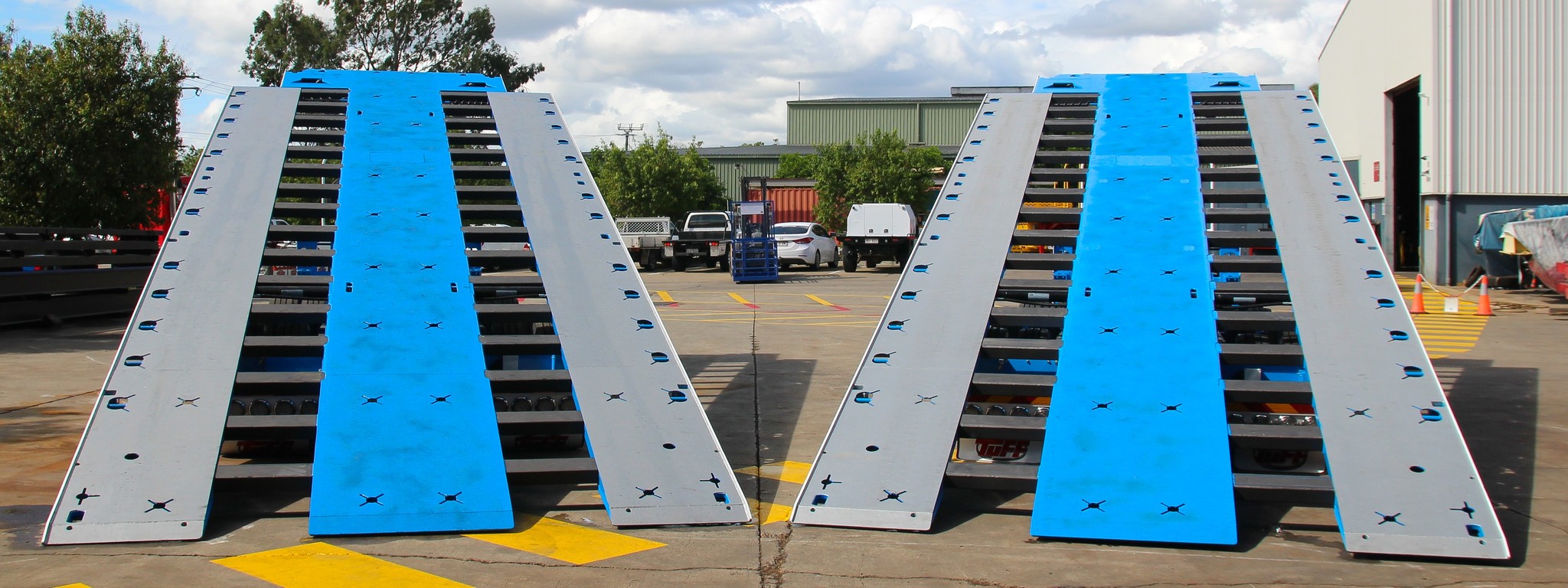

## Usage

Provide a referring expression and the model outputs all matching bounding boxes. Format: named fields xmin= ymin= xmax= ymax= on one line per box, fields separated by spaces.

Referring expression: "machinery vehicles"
xmin=729 ymin=176 xmax=779 ymax=282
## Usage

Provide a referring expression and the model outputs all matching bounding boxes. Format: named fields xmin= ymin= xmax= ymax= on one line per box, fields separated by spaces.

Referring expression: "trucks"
xmin=615 ymin=216 xmax=679 ymax=271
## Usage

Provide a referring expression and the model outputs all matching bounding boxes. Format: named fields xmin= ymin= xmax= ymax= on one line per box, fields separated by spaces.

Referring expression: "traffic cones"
xmin=1473 ymin=276 xmax=1495 ymax=316
xmin=1409 ymin=275 xmax=1429 ymax=314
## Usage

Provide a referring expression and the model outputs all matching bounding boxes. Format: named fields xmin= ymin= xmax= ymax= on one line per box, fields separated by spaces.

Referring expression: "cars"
xmin=767 ymin=222 xmax=839 ymax=272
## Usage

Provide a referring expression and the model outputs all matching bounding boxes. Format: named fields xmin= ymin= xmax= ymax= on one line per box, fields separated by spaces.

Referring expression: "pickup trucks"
xmin=836 ymin=202 xmax=922 ymax=273
xmin=661 ymin=211 xmax=745 ymax=272
